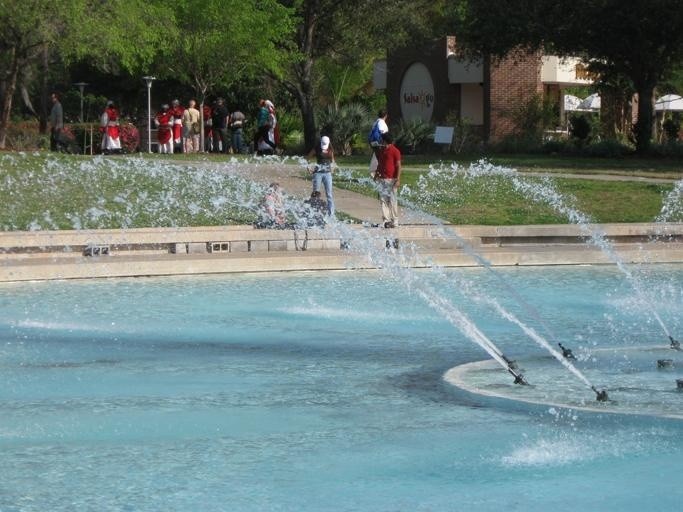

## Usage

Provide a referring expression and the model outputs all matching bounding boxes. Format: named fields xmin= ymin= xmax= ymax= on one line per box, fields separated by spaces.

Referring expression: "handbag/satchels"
xmin=368 ymin=121 xmax=384 ymax=150
xmin=193 ymin=122 xmax=200 ymax=133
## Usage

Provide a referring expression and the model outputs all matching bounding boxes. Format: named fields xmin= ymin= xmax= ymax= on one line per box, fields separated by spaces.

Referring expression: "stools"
xmin=84 ymin=120 xmax=102 ymax=155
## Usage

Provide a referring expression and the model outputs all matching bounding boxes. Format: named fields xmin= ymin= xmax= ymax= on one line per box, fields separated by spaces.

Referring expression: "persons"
xmin=99 ymin=101 xmax=123 ymax=155
xmin=368 ymin=109 xmax=388 ymax=157
xmin=306 ymin=136 xmax=335 ymax=217
xmin=300 ymin=191 xmax=327 ymax=230
xmin=373 ymin=132 xmax=401 ymax=227
xmin=153 ymin=98 xmax=278 ymax=154
xmin=255 ymin=183 xmax=297 ymax=230
xmin=49 ymin=92 xmax=63 ymax=151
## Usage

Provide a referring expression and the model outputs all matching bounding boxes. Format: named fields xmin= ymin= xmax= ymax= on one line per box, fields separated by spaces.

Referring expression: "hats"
xmin=321 ymin=136 xmax=330 ymax=150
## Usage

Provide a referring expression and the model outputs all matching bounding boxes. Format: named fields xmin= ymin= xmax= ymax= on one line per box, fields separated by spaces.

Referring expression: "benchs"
xmin=147 ymin=129 xmax=176 ymax=153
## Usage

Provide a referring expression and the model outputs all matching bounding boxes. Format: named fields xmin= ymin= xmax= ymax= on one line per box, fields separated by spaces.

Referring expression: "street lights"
xmin=142 ymin=75 xmax=156 ymax=153
xmin=71 ymin=82 xmax=89 ymax=123
